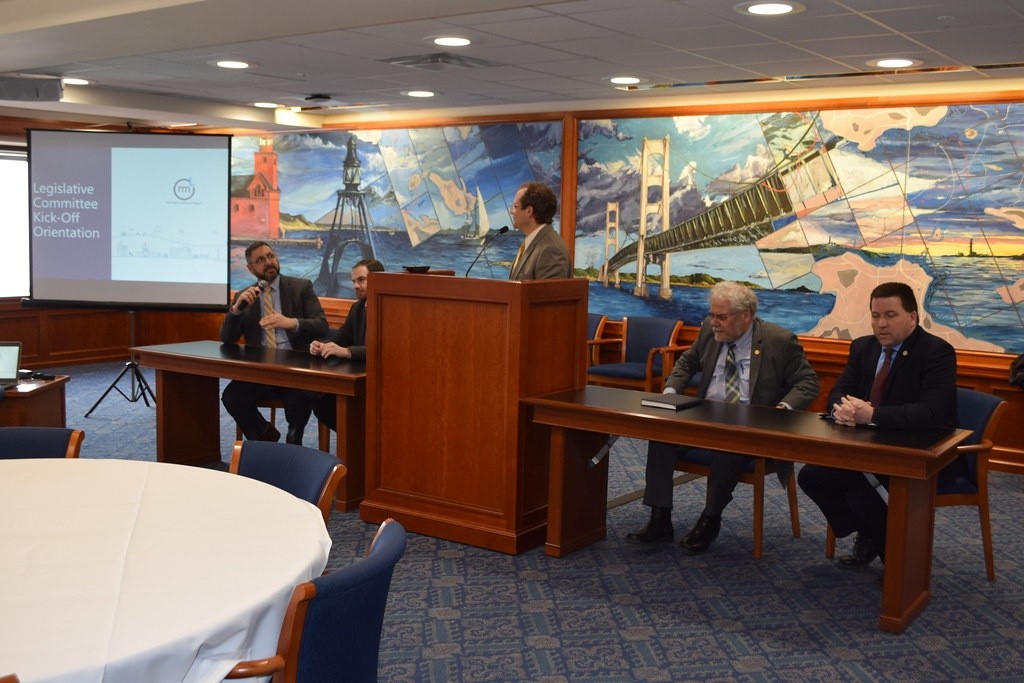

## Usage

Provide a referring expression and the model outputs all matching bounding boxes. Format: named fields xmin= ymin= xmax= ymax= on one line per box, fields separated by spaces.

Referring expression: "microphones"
xmin=466 ymin=226 xmax=509 ymax=276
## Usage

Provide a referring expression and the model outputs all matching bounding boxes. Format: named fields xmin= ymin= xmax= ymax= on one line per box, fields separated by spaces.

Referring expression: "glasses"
xmin=710 ymin=310 xmax=747 ymax=321
xmin=510 ymin=202 xmax=522 ymax=211
xmin=250 ymin=253 xmax=274 ymax=265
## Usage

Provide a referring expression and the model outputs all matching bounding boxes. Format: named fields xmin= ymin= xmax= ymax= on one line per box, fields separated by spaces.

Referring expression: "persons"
xmin=219 ymin=242 xmax=329 ymax=445
xmin=509 ymin=183 xmax=572 ymax=281
xmin=310 ymin=258 xmax=385 ymax=431
xmin=625 ymin=281 xmax=821 ymax=553
xmin=797 ymin=282 xmax=957 ymax=565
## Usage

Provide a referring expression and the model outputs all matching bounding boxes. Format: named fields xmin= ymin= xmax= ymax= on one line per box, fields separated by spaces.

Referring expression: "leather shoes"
xmin=680 ymin=513 xmax=721 ymax=554
xmin=626 ymin=514 xmax=674 ymax=546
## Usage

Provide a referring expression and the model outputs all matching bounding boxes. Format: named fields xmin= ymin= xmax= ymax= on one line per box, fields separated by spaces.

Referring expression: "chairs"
xmin=0 ymin=314 xmax=1009 ymax=683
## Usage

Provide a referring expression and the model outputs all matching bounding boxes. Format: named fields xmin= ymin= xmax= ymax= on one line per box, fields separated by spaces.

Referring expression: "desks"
xmin=0 ymin=375 xmax=70 ymax=427
xmin=127 ymin=340 xmax=367 ymax=513
xmin=518 ymin=385 xmax=975 ymax=633
xmin=0 ymin=458 xmax=332 ymax=683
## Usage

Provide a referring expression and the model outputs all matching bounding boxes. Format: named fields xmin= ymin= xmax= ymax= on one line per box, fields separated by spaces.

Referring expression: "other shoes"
xmin=255 ymin=422 xmax=280 ymax=443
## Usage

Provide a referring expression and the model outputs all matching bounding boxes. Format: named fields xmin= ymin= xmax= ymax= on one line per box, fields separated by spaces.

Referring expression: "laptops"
xmin=0 ymin=341 xmax=21 ymax=390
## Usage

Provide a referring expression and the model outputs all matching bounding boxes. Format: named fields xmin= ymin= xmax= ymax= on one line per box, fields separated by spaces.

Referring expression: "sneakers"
xmin=852 ymin=520 xmax=886 ymax=566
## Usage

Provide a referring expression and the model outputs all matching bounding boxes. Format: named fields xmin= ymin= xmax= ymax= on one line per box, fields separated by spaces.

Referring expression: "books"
xmin=641 ymin=392 xmax=702 ymax=411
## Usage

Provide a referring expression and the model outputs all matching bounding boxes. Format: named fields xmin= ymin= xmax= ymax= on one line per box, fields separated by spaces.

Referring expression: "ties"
xmin=517 ymin=240 xmax=525 ymax=263
xmin=263 ymin=286 xmax=276 ymax=349
xmin=724 ymin=342 xmax=740 ymax=403
xmin=869 ymin=349 xmax=898 ymax=408
